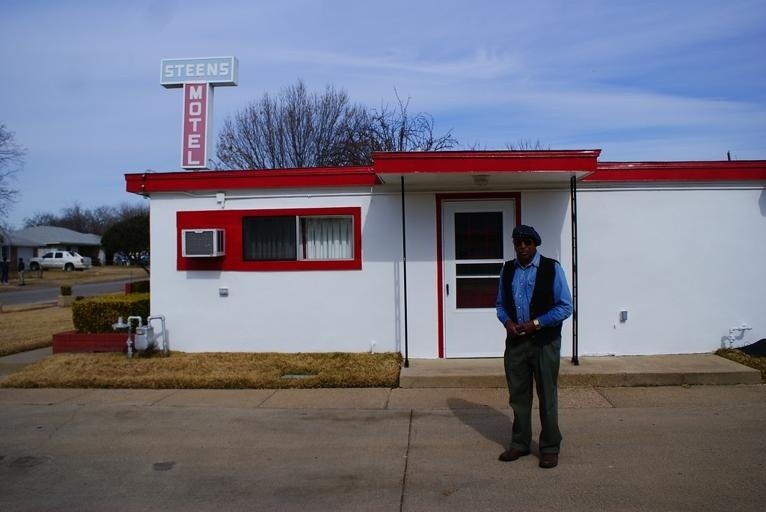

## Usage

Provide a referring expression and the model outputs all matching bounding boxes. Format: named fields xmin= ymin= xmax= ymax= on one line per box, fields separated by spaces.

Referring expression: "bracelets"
xmin=532 ymin=319 xmax=541 ymax=330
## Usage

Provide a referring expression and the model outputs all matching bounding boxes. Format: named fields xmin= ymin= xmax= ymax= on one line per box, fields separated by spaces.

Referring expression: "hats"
xmin=513 ymin=225 xmax=541 ymax=246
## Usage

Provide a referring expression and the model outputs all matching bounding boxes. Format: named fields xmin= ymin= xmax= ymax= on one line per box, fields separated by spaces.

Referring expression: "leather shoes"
xmin=539 ymin=453 xmax=558 ymax=467
xmin=500 ymin=448 xmax=530 ymax=461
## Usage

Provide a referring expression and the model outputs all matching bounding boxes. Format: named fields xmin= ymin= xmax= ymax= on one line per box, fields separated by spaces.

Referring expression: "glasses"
xmin=514 ymin=240 xmax=532 ymax=247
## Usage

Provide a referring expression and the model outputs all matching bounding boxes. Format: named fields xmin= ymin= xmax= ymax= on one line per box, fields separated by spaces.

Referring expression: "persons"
xmin=494 ymin=225 xmax=574 ymax=469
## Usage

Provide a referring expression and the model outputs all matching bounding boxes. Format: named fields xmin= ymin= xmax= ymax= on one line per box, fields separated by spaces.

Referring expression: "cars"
xmin=113 ymin=250 xmax=150 ymax=267
xmin=28 ymin=251 xmax=92 ymax=272
